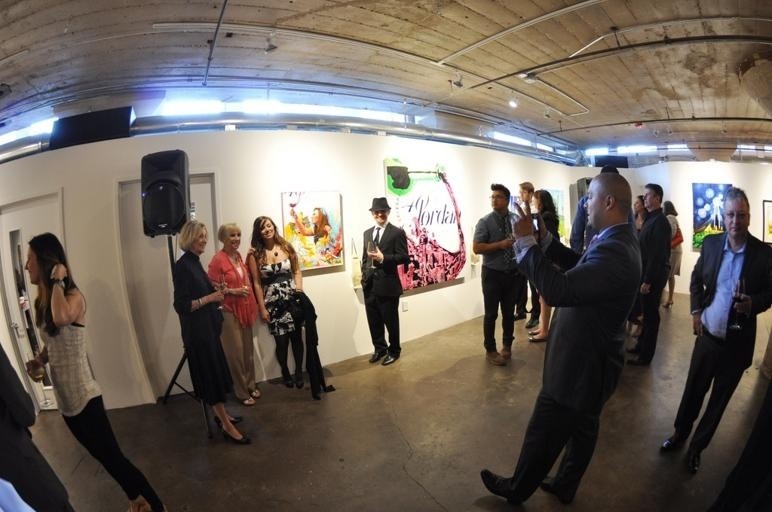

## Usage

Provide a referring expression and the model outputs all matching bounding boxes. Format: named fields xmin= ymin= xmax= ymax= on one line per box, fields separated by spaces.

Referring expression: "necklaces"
xmin=263 ymin=244 xmax=278 ymax=257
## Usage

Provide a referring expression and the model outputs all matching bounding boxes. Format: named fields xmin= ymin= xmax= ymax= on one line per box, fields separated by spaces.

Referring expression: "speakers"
xmin=591 ymin=155 xmax=629 ymax=168
xmin=141 ymin=149 xmax=191 ymax=238
xmin=578 ymin=177 xmax=593 ymax=202
xmin=49 ymin=106 xmax=137 ymax=151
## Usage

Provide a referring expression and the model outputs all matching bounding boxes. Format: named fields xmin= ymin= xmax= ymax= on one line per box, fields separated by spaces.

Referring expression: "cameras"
xmin=532 ymin=213 xmax=540 ymax=232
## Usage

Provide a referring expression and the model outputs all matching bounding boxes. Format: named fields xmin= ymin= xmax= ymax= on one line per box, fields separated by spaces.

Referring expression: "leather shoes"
xmin=481 ymin=469 xmax=523 ymax=504
xmin=514 ymin=313 xmax=526 ymax=321
xmin=526 ymin=318 xmax=539 ymax=328
xmin=663 ymin=434 xmax=688 ymax=448
xmin=370 ymin=350 xmax=387 ymax=362
xmin=383 ymin=355 xmax=396 ymax=364
xmin=686 ymin=446 xmax=700 ymax=473
xmin=625 ymin=346 xmax=640 ymax=353
xmin=627 ymin=356 xmax=650 ymax=366
xmin=528 ymin=331 xmax=548 ymax=342
xmin=243 ymin=397 xmax=256 ymax=405
xmin=250 ymin=390 xmax=261 ymax=397
xmin=281 ymin=370 xmax=294 ymax=388
xmin=296 ymin=376 xmax=304 ymax=388
xmin=541 ymin=476 xmax=574 ymax=504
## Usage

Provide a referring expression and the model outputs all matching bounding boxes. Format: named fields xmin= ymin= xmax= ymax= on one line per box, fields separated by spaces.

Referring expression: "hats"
xmin=369 ymin=198 xmax=392 ymax=211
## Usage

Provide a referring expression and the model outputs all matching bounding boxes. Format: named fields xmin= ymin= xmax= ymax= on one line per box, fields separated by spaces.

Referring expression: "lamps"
xmin=448 ymin=67 xmax=466 ymax=89
xmin=261 ymin=28 xmax=280 ymax=53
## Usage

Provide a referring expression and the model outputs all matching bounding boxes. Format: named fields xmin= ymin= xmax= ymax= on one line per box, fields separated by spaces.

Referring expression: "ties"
xmin=374 ymin=227 xmax=380 ymax=246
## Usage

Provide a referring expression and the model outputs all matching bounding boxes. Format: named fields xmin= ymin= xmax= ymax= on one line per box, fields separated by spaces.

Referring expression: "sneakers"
xmin=486 ymin=352 xmax=507 ymax=365
xmin=501 ymin=349 xmax=511 ymax=359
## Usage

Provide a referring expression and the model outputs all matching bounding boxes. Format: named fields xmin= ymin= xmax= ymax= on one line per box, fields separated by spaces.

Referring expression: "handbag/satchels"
xmin=669 ymin=228 xmax=683 ymax=247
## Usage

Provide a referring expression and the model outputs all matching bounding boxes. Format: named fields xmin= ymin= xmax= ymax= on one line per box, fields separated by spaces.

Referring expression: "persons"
xmin=624 ymin=183 xmax=683 ymax=365
xmin=661 ymin=187 xmax=772 ymax=474
xmin=291 ymin=208 xmax=333 ymax=267
xmin=473 ymin=183 xmax=523 ymax=364
xmin=0 ymin=343 xmax=76 ymax=511
xmin=207 ymin=223 xmax=261 ymax=406
xmin=25 ymin=232 xmax=167 ymax=512
xmin=172 ymin=220 xmax=252 ymax=445
xmin=513 ymin=181 xmax=560 ymax=341
xmin=569 ymin=165 xmax=619 ymax=255
xmin=361 ymin=198 xmax=409 ymax=365
xmin=694 ymin=192 xmax=724 ymax=233
xmin=481 ymin=172 xmax=643 ymax=506
xmin=248 ymin=216 xmax=304 ymax=389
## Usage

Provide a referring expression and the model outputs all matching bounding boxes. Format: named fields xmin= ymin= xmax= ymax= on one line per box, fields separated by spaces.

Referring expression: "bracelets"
xmin=198 ymin=298 xmax=202 ymax=308
xmin=227 ymin=289 xmax=232 ymax=294
xmin=296 ymin=289 xmax=302 ymax=292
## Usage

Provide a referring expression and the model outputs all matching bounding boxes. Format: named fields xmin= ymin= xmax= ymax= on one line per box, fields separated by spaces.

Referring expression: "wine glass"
xmin=24 ymin=357 xmax=55 ymax=407
xmin=237 ymin=274 xmax=253 ymax=304
xmin=367 ymin=241 xmax=378 ymax=270
xmin=729 ymin=276 xmax=745 ymax=330
xmin=216 ymin=273 xmax=227 ymax=311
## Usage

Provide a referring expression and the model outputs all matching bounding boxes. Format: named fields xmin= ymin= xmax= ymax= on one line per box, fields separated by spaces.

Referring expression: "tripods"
xmin=163 ymin=235 xmax=214 ymax=439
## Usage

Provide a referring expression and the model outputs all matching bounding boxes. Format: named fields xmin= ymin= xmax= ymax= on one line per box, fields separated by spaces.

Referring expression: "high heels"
xmin=221 ymin=427 xmax=251 ymax=444
xmin=663 ymin=301 xmax=673 ymax=308
xmin=214 ymin=415 xmax=243 ymax=427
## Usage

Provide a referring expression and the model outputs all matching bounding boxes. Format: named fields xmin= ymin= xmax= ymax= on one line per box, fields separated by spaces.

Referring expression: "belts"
xmin=702 ymin=327 xmax=725 ymax=346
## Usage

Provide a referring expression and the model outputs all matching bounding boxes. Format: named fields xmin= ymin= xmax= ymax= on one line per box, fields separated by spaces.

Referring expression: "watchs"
xmin=53 ymin=279 xmax=66 ymax=288
